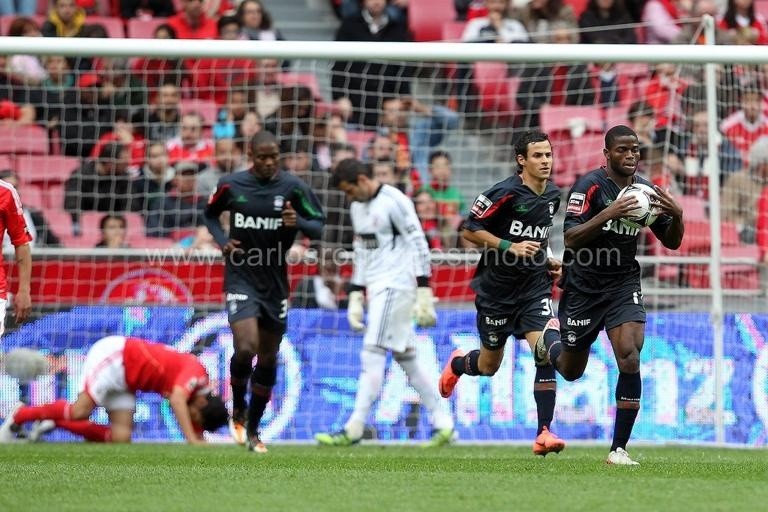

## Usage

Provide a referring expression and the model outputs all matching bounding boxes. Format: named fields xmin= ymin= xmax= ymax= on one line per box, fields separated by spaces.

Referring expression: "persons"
xmin=313 ymin=158 xmax=460 ymax=450
xmin=0 ymin=178 xmax=36 ymax=333
xmin=533 ymin=125 xmax=685 ymax=465
xmin=203 ymin=130 xmax=328 ymax=454
xmin=0 ymin=335 xmax=229 ymax=446
xmin=437 ymin=129 xmax=566 ymax=454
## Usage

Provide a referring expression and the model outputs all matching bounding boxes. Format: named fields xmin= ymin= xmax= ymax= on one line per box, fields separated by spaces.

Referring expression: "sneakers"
xmin=532 ymin=427 xmax=568 ymax=460
xmin=247 ymin=435 xmax=272 ymax=455
xmin=309 ymin=423 xmax=365 ymax=450
xmin=530 ymin=315 xmax=563 ymax=365
xmin=421 ymin=425 xmax=462 ymax=450
xmin=0 ymin=398 xmax=25 ymax=445
xmin=606 ymin=444 xmax=643 ymax=469
xmin=226 ymin=415 xmax=248 ymax=447
xmin=438 ymin=347 xmax=467 ymax=401
xmin=27 ymin=414 xmax=57 ymax=446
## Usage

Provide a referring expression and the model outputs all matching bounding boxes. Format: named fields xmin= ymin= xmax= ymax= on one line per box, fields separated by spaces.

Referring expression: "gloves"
xmin=346 ymin=288 xmax=368 ymax=335
xmin=409 ymin=287 xmax=442 ymax=330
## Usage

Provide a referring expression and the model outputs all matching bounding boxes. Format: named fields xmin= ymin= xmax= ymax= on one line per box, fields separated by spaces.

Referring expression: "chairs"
xmin=1 ymin=1 xmax=766 ymax=291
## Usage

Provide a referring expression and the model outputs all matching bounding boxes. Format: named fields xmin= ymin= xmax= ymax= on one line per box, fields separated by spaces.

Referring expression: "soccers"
xmin=616 ymin=183 xmax=663 ymax=230
